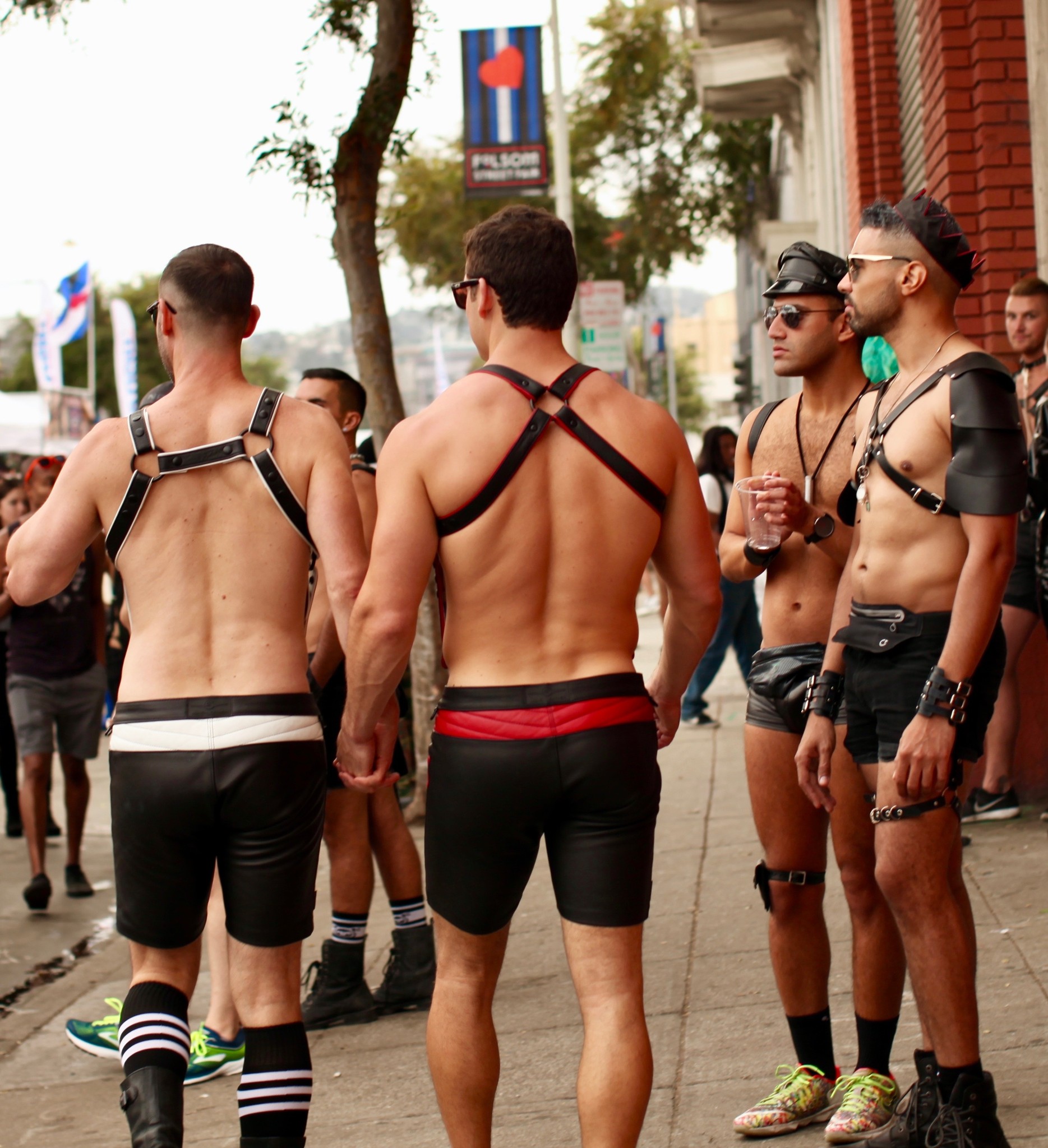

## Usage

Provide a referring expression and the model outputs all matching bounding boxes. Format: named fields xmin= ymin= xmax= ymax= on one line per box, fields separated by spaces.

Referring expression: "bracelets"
xmin=799 ymin=670 xmax=847 ymax=723
xmin=742 ymin=540 xmax=781 ymax=567
xmin=916 ymin=664 xmax=973 ymax=726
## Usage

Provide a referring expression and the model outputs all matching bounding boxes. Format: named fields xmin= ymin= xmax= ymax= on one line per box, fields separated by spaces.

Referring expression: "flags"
xmin=641 ymin=310 xmax=671 ymax=363
xmin=53 ymin=260 xmax=89 ymax=347
xmin=607 ymin=367 xmax=635 ymax=392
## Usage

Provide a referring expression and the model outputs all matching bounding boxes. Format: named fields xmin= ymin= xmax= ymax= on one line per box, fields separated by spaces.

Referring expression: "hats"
xmin=760 ymin=242 xmax=848 ymax=299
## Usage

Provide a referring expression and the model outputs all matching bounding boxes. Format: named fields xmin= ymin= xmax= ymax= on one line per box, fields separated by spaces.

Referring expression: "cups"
xmin=734 ymin=474 xmax=785 ymax=550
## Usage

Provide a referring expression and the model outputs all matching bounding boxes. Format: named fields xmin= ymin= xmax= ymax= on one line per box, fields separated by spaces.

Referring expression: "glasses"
xmin=845 ymin=253 xmax=911 ymax=281
xmin=146 ymin=299 xmax=176 ymax=326
xmin=451 ymin=277 xmax=500 ymax=311
xmin=763 ymin=304 xmax=848 ymax=331
xmin=24 ymin=455 xmax=67 ymax=489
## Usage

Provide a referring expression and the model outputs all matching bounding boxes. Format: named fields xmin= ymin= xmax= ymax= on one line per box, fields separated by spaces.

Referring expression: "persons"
xmin=4 ymin=244 xmax=400 ymax=1147
xmin=958 ymin=275 xmax=1048 ymax=827
xmin=793 ymin=192 xmax=1030 ymax=1148
xmin=336 ymin=203 xmax=723 ymax=1147
xmin=1 ymin=365 xmax=437 ymax=1087
xmin=677 ymin=425 xmax=764 ymax=730
xmin=718 ymin=239 xmax=909 ymax=1145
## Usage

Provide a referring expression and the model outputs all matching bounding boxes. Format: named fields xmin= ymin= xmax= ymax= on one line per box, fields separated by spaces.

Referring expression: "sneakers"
xmin=959 ymin=786 xmax=1021 ymax=823
xmin=182 ymin=1022 xmax=245 ymax=1086
xmin=66 ymin=997 xmax=123 ymax=1060
xmin=733 ymin=1064 xmax=846 ymax=1136
xmin=823 ymin=1067 xmax=906 ymax=1142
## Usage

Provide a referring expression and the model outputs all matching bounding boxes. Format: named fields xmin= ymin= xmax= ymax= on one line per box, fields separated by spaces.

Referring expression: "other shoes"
xmin=23 ymin=876 xmax=51 ymax=910
xmin=63 ymin=866 xmax=92 ymax=898
xmin=685 ymin=712 xmax=719 ymax=730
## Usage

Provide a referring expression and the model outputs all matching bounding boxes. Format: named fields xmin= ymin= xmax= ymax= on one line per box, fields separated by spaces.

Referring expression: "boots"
xmin=372 ymin=918 xmax=437 ymax=1015
xmin=119 ymin=1066 xmax=183 ymax=1148
xmin=241 ymin=1136 xmax=306 ymax=1148
xmin=300 ymin=940 xmax=377 ymax=1031
xmin=837 ymin=1048 xmax=938 ymax=1148
xmin=925 ymin=1071 xmax=1011 ymax=1148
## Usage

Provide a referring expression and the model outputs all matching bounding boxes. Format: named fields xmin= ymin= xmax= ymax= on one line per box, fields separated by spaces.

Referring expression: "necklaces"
xmin=1020 ymin=353 xmax=1048 ymax=369
xmin=856 ymin=330 xmax=961 ymax=512
xmin=795 ymin=377 xmax=872 ymax=505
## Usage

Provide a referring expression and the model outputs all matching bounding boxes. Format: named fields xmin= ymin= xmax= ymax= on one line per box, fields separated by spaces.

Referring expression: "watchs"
xmin=803 ymin=511 xmax=835 ymax=544
